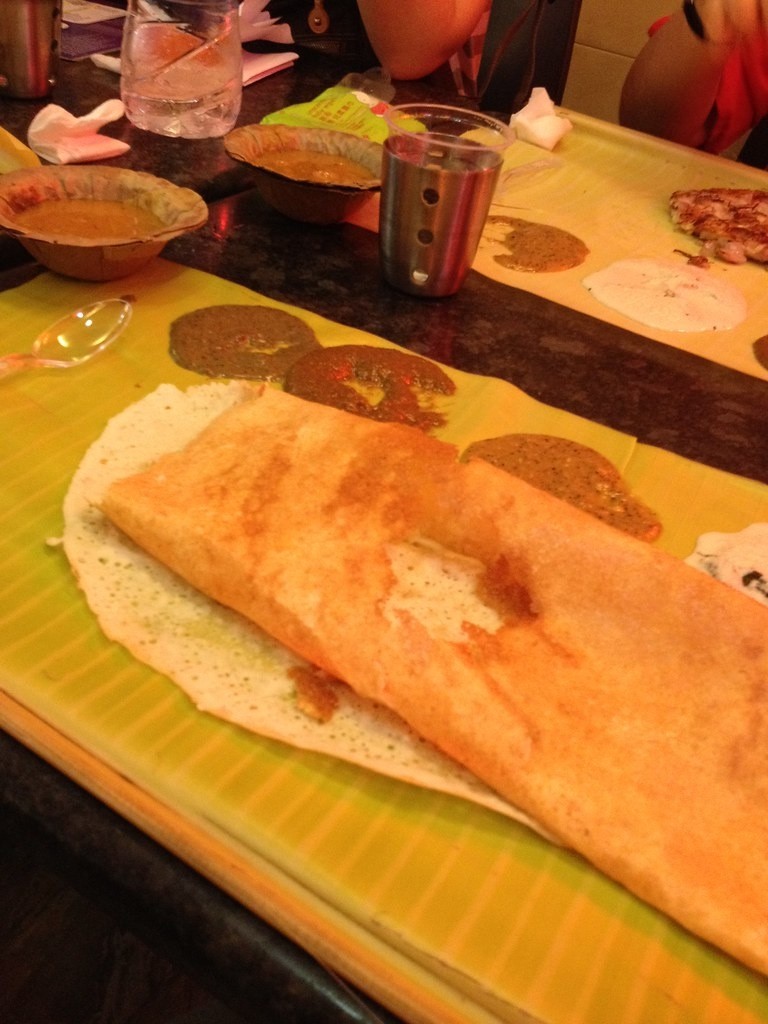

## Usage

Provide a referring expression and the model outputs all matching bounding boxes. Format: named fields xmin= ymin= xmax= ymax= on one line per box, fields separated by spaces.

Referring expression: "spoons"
xmin=0 ymin=298 xmax=133 ymax=378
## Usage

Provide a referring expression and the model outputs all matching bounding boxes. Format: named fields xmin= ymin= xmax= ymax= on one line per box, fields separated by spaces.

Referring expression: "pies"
xmin=670 ymin=186 xmax=768 ymax=267
xmin=58 ymin=380 xmax=768 ymax=979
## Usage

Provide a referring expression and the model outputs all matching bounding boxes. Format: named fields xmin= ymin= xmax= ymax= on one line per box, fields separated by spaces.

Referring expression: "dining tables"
xmin=0 ymin=0 xmax=768 ymax=1024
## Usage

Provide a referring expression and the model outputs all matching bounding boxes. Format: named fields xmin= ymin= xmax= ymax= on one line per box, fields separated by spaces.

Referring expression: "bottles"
xmin=120 ymin=1 xmax=242 ymax=139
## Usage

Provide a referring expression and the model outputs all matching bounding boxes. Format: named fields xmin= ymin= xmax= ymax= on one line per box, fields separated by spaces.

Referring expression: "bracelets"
xmin=683 ymin=0 xmax=707 ymax=40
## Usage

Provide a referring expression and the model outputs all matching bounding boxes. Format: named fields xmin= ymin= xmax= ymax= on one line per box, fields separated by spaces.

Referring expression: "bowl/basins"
xmin=0 ymin=166 xmax=209 ymax=280
xmin=224 ymin=124 xmax=384 ymax=222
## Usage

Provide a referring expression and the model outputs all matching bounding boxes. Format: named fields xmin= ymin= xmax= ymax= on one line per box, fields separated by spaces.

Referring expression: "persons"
xmin=241 ymin=0 xmax=582 ymax=110
xmin=619 ymin=1 xmax=768 ymax=172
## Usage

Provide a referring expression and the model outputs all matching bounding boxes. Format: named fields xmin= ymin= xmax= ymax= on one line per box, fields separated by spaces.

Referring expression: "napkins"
xmin=507 ymin=86 xmax=573 ymax=151
xmin=27 ymin=98 xmax=131 ymax=166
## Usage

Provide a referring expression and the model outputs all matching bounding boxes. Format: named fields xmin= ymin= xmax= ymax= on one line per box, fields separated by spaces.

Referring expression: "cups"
xmin=385 ymin=103 xmax=514 ymax=168
